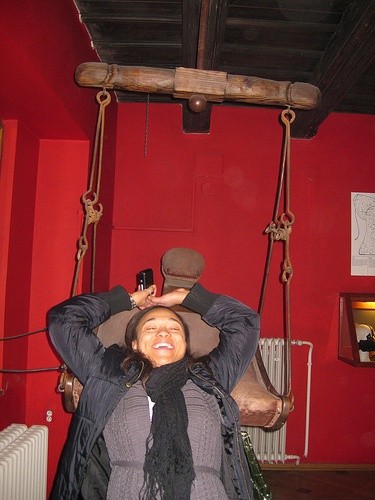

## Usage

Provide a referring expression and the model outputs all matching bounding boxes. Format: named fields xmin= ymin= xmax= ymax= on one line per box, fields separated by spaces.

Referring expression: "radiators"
xmin=0 ymin=425 xmax=48 ymax=500
xmin=0 ymin=423 xmax=29 ymax=451
xmin=236 ymin=337 xmax=314 ymax=465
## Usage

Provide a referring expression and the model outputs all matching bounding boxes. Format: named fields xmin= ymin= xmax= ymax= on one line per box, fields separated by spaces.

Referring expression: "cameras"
xmin=140 ymin=269 xmax=154 ymax=290
